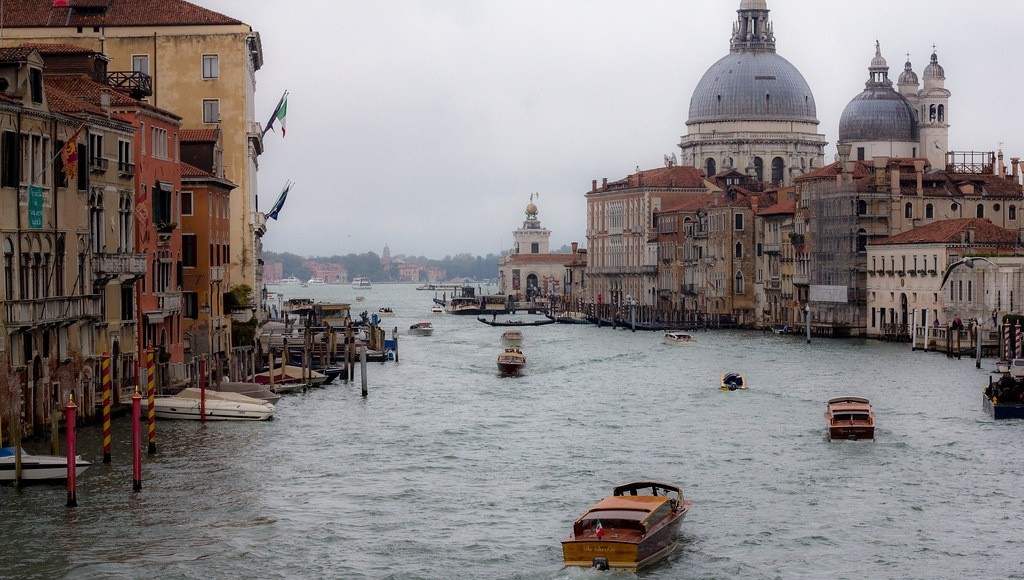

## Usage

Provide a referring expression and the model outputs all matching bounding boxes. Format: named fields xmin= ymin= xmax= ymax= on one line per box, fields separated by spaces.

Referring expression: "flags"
xmin=269 ymin=187 xmax=288 ymax=221
xmin=63 ymin=131 xmax=77 ymax=182
xmin=276 ymin=100 xmax=287 ymax=138
xmin=268 ymin=107 xmax=279 ymax=133
xmin=596 ymin=517 xmax=604 ymax=538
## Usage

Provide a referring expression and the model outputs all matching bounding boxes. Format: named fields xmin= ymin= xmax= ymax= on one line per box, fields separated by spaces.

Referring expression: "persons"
xmin=504 ymin=347 xmax=522 ymax=360
xmin=932 ymin=308 xmax=998 ymax=339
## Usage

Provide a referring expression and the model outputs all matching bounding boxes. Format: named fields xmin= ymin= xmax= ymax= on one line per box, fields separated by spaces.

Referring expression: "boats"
xmin=281 ymin=276 xmax=490 ymax=291
xmin=500 ymin=330 xmax=525 ymax=348
xmin=378 ymin=307 xmax=396 ymax=318
xmin=823 ymin=396 xmax=876 ymax=443
xmin=559 ymin=480 xmax=692 ymax=575
xmin=448 ymin=295 xmax=507 ymax=314
xmin=663 ymin=333 xmax=698 ymax=347
xmin=407 ymin=322 xmax=434 ymax=337
xmin=445 ymin=298 xmax=480 ymax=313
xmin=983 ymin=370 xmax=1024 ymax=421
xmin=431 ymin=305 xmax=442 ymax=313
xmin=356 ymin=296 xmax=365 ymax=303
xmin=720 ymin=370 xmax=746 ymax=392
xmin=0 ymin=447 xmax=90 ymax=483
xmin=557 ymin=311 xmax=590 ymax=325
xmin=496 ymin=348 xmax=528 ymax=375
xmin=119 ymin=298 xmax=351 ymax=421
xmin=1011 ymin=359 xmax=1024 ymax=380
